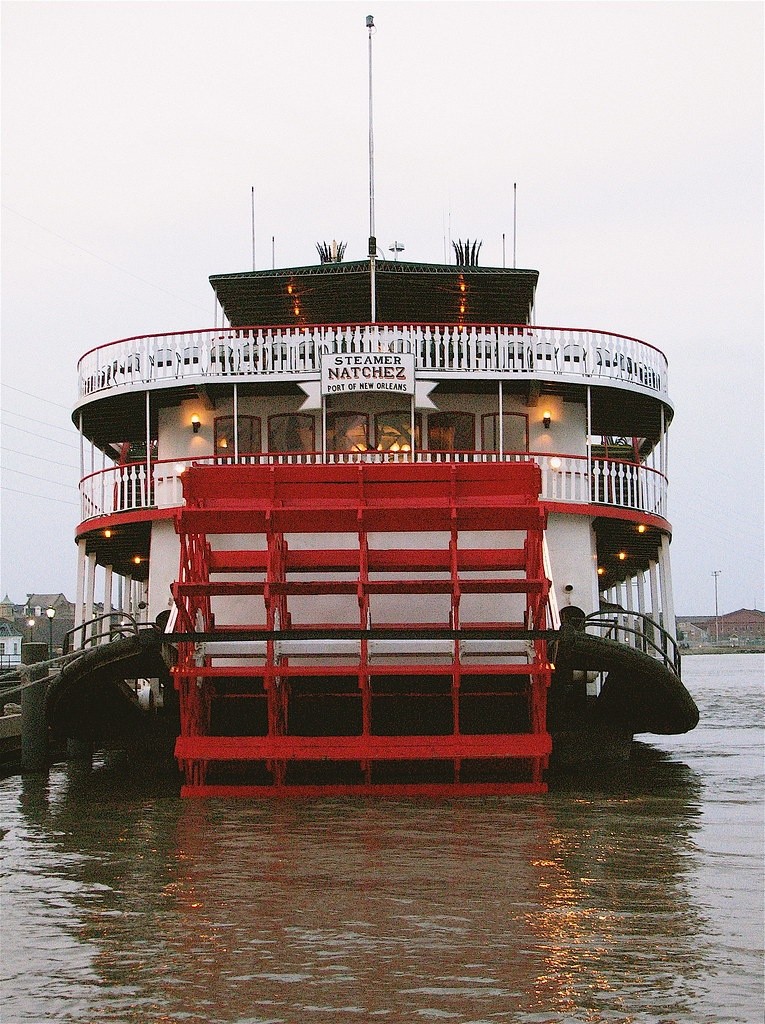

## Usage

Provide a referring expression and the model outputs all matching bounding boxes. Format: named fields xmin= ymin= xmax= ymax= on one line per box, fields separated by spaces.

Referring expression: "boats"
xmin=42 ymin=17 xmax=701 ymax=796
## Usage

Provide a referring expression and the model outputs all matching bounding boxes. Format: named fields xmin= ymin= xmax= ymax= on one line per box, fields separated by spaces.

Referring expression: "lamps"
xmin=190 ymin=413 xmax=201 ymax=433
xmin=543 ymin=410 xmax=551 ymax=428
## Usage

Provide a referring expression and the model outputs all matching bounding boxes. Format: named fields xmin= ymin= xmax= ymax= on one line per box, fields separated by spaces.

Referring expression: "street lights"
xmin=28 ymin=619 xmax=35 ymax=643
xmin=45 ymin=604 xmax=56 ymax=669
xmin=712 ymin=570 xmax=722 ymax=646
